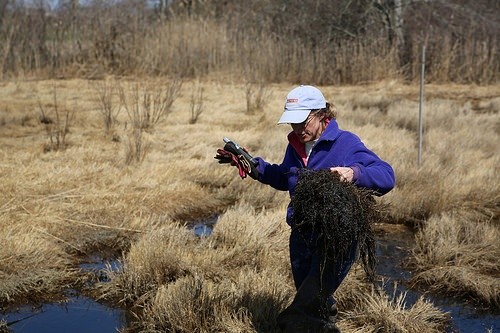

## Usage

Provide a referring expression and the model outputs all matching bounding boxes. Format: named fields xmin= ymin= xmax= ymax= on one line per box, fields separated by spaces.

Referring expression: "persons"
xmin=214 ymin=85 xmax=395 ymax=333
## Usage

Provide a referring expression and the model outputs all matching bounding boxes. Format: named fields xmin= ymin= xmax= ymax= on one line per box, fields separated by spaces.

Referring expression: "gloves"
xmin=217 ymin=138 xmax=259 ymax=177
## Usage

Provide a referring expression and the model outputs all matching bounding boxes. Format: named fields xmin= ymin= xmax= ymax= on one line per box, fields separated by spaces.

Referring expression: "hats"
xmin=279 ymin=85 xmax=328 ymax=124
